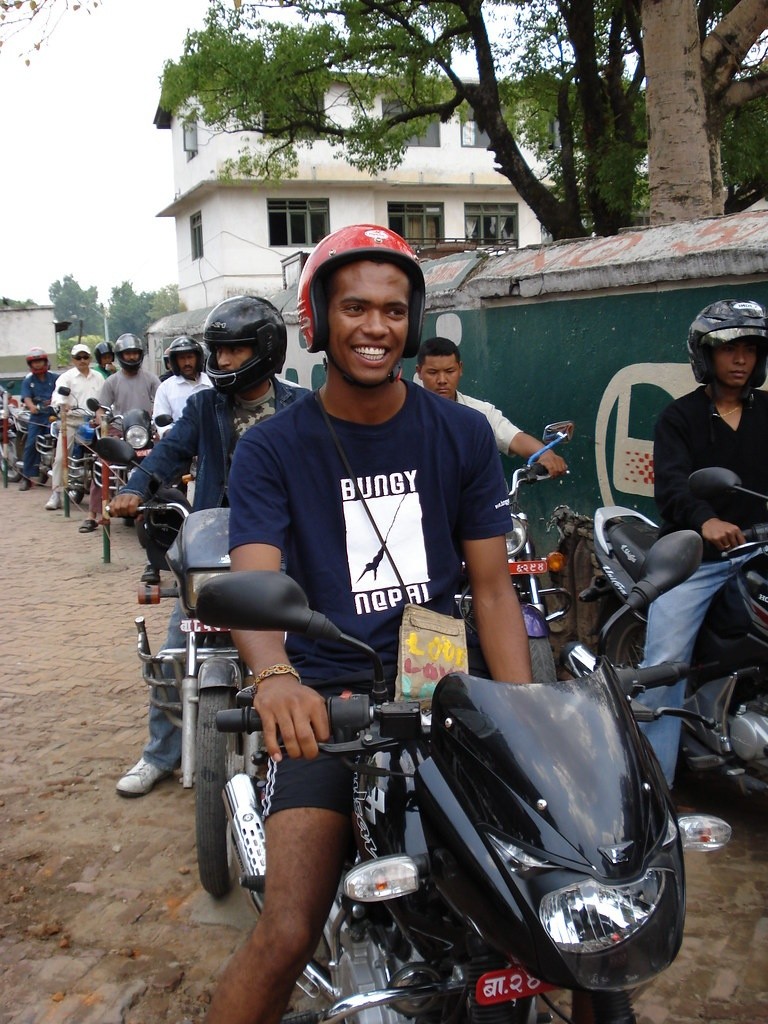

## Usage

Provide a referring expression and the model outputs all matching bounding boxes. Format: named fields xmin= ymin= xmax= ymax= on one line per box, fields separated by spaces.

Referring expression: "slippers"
xmin=79 ymin=520 xmax=98 ymax=532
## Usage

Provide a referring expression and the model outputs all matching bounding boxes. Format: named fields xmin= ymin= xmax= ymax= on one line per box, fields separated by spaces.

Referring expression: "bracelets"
xmin=251 ymin=664 xmax=301 ymax=690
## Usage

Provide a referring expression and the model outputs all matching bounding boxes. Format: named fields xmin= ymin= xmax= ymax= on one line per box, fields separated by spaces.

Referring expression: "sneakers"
xmin=45 ymin=495 xmax=61 ymax=509
xmin=115 ymin=757 xmax=173 ymax=797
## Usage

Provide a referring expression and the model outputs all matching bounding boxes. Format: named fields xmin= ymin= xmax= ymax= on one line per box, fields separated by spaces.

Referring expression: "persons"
xmin=107 ymin=295 xmax=313 ymax=798
xmin=204 ymin=223 xmax=532 ymax=1024
xmin=416 ymin=336 xmax=567 ymax=479
xmin=7 ymin=393 xmax=18 ymax=408
xmin=19 ymin=347 xmax=60 ymax=491
xmin=159 ymin=349 xmax=174 ymax=380
xmin=634 ymin=299 xmax=768 ymax=796
xmin=80 ymin=332 xmax=161 ymax=533
xmin=93 ymin=341 xmax=118 ymax=378
xmin=45 ymin=344 xmax=106 ymax=509
xmin=153 ymin=337 xmax=214 ymax=440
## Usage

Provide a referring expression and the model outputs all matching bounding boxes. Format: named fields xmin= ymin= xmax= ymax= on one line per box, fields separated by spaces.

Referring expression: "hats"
xmin=71 ymin=344 xmax=91 ymax=355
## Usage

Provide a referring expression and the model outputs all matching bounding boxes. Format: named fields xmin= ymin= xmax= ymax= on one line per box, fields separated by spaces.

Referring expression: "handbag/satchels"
xmin=393 ymin=603 xmax=469 ymax=710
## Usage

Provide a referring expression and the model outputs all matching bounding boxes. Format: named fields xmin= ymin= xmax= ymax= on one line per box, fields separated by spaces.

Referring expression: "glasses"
xmin=73 ymin=355 xmax=89 ymax=360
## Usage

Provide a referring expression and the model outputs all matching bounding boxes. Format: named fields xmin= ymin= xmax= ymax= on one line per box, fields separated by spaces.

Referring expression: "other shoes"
xmin=140 ymin=564 xmax=161 ymax=583
xmin=19 ymin=479 xmax=31 ymax=491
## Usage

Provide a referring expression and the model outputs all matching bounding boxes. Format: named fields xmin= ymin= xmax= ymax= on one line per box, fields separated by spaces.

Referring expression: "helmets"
xmin=114 ymin=333 xmax=144 ymax=372
xmin=202 ymin=296 xmax=288 ymax=395
xmin=94 ymin=342 xmax=115 ymax=365
xmin=686 ymin=299 xmax=768 ymax=388
xmin=297 ymin=224 xmax=425 ymax=358
xmin=163 ymin=348 xmax=170 ymax=370
xmin=25 ymin=348 xmax=50 ymax=375
xmin=168 ymin=336 xmax=205 ymax=376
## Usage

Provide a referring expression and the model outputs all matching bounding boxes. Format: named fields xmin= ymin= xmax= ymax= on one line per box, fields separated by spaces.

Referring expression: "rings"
xmin=725 ymin=544 xmax=731 ymax=549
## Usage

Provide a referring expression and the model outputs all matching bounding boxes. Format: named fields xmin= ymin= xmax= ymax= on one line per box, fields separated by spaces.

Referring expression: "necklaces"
xmin=720 ymin=408 xmax=737 ymax=417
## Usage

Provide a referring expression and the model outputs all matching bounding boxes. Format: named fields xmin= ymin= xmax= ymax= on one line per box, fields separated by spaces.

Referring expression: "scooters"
xmin=191 ymin=529 xmax=730 ymax=1024
xmin=451 ymin=417 xmax=581 ymax=686
xmin=0 ymin=386 xmax=296 ymax=897
xmin=562 ymin=464 xmax=768 ymax=810
